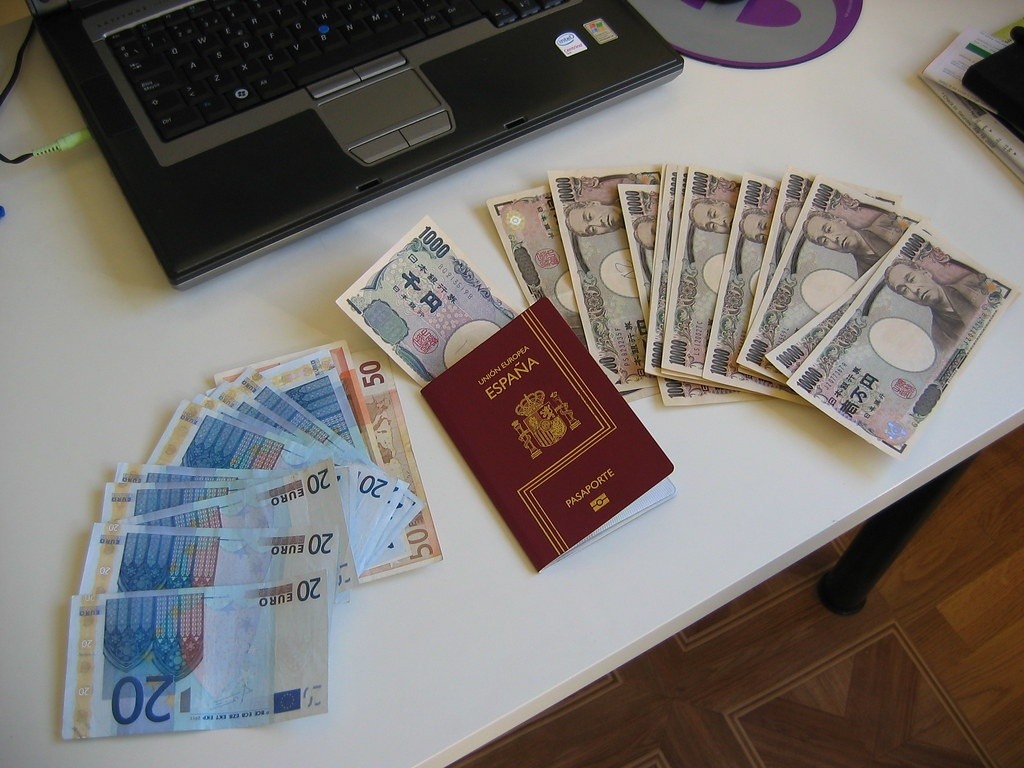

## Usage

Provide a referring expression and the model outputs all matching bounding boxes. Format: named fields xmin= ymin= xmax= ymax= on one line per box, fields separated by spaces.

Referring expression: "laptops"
xmin=25 ymin=0 xmax=683 ymax=290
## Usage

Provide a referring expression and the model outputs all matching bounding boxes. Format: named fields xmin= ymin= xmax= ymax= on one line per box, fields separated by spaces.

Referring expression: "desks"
xmin=0 ymin=0 xmax=1024 ymax=767
